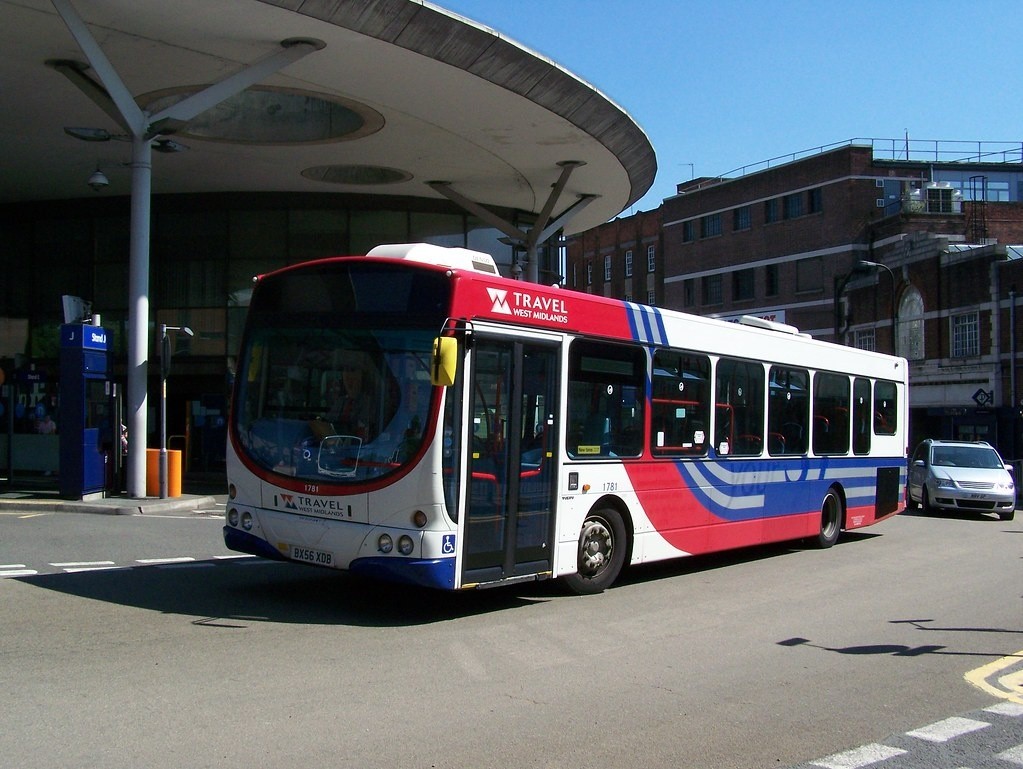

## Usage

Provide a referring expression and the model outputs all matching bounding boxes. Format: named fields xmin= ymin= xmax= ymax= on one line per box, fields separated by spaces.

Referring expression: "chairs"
xmin=366 ymin=392 xmax=896 ymax=485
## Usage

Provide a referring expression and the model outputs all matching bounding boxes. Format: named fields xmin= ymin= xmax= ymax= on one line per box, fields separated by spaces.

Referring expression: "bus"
xmin=224 ymin=243 xmax=908 ymax=597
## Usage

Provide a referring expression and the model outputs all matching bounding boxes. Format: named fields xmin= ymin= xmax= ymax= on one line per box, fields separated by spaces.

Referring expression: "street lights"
xmin=857 ymin=260 xmax=896 ymax=356
xmin=159 ymin=324 xmax=195 ymax=498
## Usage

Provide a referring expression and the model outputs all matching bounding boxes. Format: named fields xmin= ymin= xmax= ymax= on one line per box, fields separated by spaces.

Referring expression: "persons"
xmin=37 ymin=414 xmax=56 ymax=434
xmin=120 ymin=423 xmax=128 ymax=455
xmin=318 ymin=360 xmax=375 ymax=445
xmin=47 ymin=394 xmax=59 ymax=420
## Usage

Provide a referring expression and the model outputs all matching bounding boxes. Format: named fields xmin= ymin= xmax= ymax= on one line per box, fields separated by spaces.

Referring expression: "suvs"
xmin=906 ymin=438 xmax=1015 ymax=520
